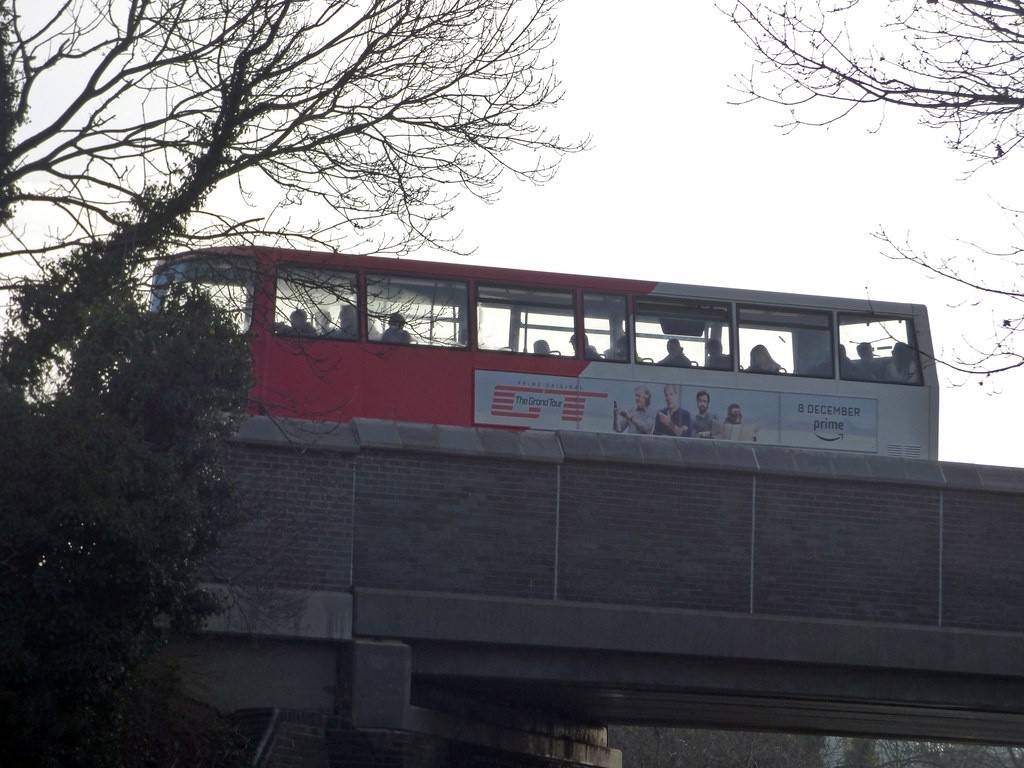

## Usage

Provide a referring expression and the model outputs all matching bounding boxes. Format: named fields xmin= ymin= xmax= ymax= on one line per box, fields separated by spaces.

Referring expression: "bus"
xmin=143 ymin=245 xmax=938 ymax=462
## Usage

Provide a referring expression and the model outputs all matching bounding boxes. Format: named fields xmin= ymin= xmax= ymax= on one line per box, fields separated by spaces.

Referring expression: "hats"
xmin=569 ymin=333 xmax=588 ymax=345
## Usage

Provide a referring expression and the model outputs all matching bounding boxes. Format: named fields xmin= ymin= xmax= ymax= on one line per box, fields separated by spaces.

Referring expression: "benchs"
xmin=852 ymin=356 xmax=893 ymax=382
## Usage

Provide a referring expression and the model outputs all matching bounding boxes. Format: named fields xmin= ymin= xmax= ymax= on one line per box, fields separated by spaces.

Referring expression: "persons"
xmin=616 ymin=334 xmax=642 ymax=364
xmin=534 ymin=339 xmax=551 ymax=355
xmin=706 ymin=339 xmax=734 ymax=371
xmin=282 ymin=309 xmax=318 ymax=338
xmin=837 ymin=342 xmax=921 ymax=386
xmin=381 ymin=314 xmax=411 ymax=346
xmin=656 ymin=339 xmax=690 ymax=367
xmin=743 ymin=344 xmax=780 ymax=374
xmin=614 ymin=384 xmax=762 ymax=442
xmin=570 ymin=333 xmax=603 ymax=360
